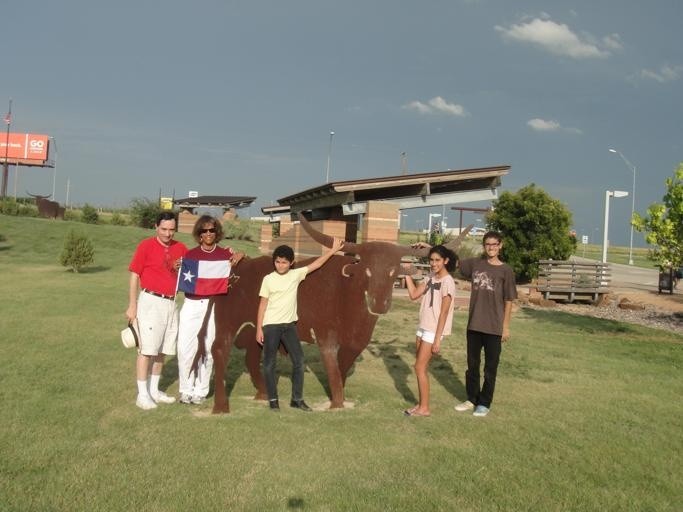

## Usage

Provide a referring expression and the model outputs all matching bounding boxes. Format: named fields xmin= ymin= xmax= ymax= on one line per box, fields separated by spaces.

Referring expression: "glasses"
xmin=484 ymin=241 xmax=502 ymax=247
xmin=197 ymin=227 xmax=218 ymax=234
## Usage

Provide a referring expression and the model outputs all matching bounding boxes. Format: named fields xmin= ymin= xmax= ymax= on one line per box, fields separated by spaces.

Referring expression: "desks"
xmin=413 ymin=263 xmax=432 ymax=271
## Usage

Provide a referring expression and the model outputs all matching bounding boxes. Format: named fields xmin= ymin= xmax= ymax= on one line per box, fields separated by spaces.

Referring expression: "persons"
xmin=172 ymin=216 xmax=245 ymax=407
xmin=254 ymin=235 xmax=344 ymax=411
xmin=411 ymin=231 xmax=518 ymax=416
xmin=404 ymin=246 xmax=459 ymax=416
xmin=126 ymin=212 xmax=233 ymax=410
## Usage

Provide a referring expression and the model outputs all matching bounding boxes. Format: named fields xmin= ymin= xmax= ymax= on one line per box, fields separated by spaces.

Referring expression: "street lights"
xmin=325 ymin=130 xmax=336 ymax=184
xmin=601 ymin=189 xmax=631 ymax=285
xmin=609 ymin=147 xmax=638 ymax=263
xmin=428 ymin=213 xmax=441 ymax=244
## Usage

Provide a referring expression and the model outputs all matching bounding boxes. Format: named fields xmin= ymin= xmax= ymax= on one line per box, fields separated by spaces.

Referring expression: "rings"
xmin=233 ymin=259 xmax=236 ymax=263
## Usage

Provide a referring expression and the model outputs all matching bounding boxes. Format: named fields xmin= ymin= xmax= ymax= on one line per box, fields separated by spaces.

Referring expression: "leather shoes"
xmin=290 ymin=399 xmax=313 ymax=412
xmin=269 ymin=399 xmax=280 ymax=411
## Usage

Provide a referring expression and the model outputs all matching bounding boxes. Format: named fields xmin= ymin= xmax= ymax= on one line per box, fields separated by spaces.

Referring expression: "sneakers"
xmin=135 ymin=391 xmax=159 ymax=411
xmin=454 ymin=400 xmax=476 ymax=411
xmin=191 ymin=393 xmax=207 ymax=405
xmin=147 ymin=388 xmax=177 ymax=405
xmin=472 ymin=403 xmax=490 ymax=418
xmin=179 ymin=393 xmax=192 ymax=405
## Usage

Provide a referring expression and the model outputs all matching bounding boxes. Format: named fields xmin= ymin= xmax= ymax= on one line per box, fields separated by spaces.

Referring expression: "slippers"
xmin=404 ymin=406 xmax=431 ymax=417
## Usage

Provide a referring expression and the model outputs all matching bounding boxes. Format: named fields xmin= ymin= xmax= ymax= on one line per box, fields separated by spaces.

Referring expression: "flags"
xmin=176 ymin=258 xmax=231 ymax=296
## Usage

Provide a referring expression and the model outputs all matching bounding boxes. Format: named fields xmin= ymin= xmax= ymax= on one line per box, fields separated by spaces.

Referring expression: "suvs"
xmin=462 ymin=228 xmax=487 ymax=237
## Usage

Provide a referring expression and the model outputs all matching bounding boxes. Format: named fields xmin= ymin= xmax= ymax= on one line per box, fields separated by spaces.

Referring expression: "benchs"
xmin=397 ymin=274 xmax=422 ymax=289
xmin=522 ymin=258 xmax=612 ymax=303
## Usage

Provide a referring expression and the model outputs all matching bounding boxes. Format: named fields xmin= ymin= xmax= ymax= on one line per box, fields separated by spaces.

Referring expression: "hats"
xmin=120 ymin=316 xmax=141 ymax=354
